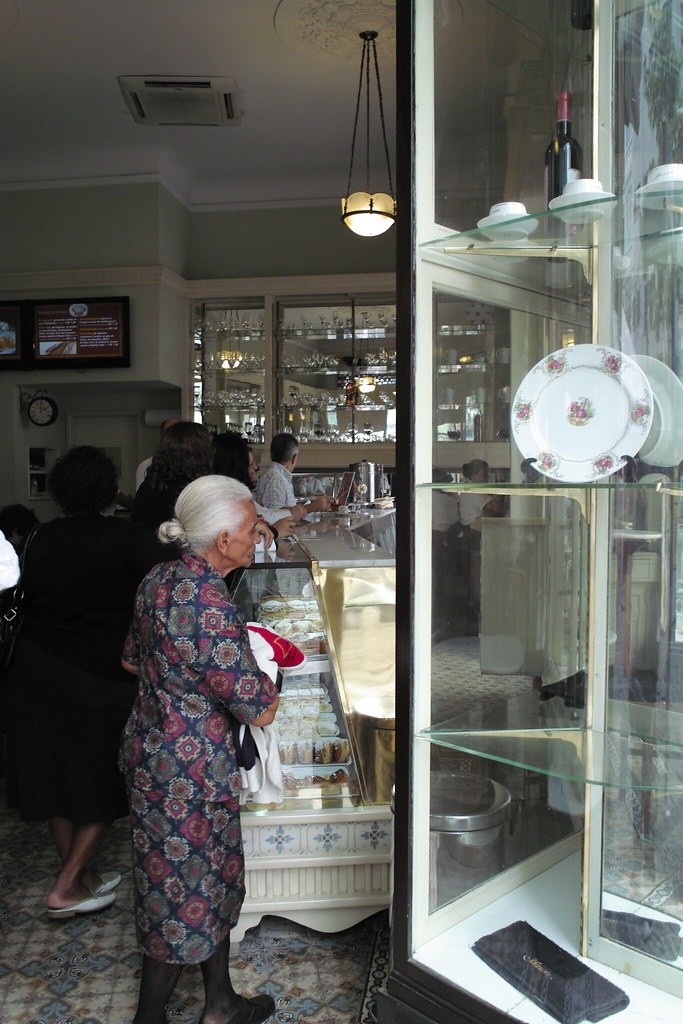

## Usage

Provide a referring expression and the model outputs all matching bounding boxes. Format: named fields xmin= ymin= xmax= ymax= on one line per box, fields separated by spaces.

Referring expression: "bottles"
xmin=544 ymin=92 xmax=582 ymax=237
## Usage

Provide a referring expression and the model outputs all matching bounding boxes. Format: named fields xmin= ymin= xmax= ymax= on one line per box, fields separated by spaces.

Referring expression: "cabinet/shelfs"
xmin=478 ymin=516 xmax=590 ymax=687
xmin=606 ymin=553 xmax=660 ymax=670
xmin=189 ymin=293 xmax=512 ymax=445
xmin=395 ymin=0 xmax=683 ymax=1024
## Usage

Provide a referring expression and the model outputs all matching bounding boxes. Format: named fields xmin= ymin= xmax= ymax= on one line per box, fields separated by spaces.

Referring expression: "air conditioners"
xmin=144 ymin=409 xmax=181 ymax=427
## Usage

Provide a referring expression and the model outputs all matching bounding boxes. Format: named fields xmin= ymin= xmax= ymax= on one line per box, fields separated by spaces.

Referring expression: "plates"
xmin=511 ymin=344 xmax=653 ymax=482
xmin=241 ymin=689 xmax=359 ymax=800
xmin=648 ymin=235 xmax=683 ymax=265
xmin=633 ymin=178 xmax=683 ymax=209
xmin=628 ymin=354 xmax=683 ymax=468
xmin=477 ymin=214 xmax=538 ymax=241
xmin=547 ymin=192 xmax=617 ymax=224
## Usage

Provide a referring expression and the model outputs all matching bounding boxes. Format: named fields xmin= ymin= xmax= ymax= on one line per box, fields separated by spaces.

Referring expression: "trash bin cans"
xmin=392 ymin=771 xmax=513 ymax=902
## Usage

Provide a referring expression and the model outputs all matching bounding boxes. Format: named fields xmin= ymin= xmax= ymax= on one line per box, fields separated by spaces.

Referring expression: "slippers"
xmin=198 ymin=993 xmax=275 ymax=1024
xmin=46 ymin=880 xmax=117 ymax=918
xmin=94 ymin=871 xmax=122 ymax=893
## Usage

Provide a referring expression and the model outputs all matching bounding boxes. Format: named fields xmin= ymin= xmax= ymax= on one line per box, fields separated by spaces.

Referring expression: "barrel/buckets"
xmin=349 ymin=460 xmax=381 ymax=503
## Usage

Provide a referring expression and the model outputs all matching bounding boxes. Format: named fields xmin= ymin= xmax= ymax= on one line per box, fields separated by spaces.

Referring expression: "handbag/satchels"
xmin=1 ymin=606 xmax=28 ymax=671
xmin=454 ymin=527 xmax=471 ymax=554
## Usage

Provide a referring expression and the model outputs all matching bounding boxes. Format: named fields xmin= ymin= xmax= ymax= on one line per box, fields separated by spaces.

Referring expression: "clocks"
xmin=28 ymin=397 xmax=58 ymax=428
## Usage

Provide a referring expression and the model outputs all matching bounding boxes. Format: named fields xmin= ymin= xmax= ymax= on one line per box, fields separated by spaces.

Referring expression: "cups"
xmin=489 ymin=202 xmax=527 ymax=218
xmin=660 ymin=226 xmax=683 ymax=239
xmin=563 ymin=180 xmax=603 ymax=195
xmin=339 ymin=506 xmax=349 ymax=514
xmin=646 ymin=164 xmax=682 ymax=182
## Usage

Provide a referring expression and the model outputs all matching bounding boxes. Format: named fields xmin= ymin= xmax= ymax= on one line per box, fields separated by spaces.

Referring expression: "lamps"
xmin=341 ymin=30 xmax=397 ymax=236
xmin=221 ymin=309 xmax=244 ymax=369
xmin=355 ymin=316 xmax=377 ymax=394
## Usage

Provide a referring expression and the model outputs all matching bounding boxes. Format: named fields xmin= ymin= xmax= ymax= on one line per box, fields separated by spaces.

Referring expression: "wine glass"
xmin=206 ymin=308 xmax=396 ymax=441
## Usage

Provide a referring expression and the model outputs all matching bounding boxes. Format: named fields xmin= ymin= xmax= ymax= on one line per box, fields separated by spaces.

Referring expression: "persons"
xmin=0 ymin=444 xmax=128 ymax=919
xmin=118 ymin=475 xmax=279 ymax=1024
xmin=130 ymin=419 xmax=334 ymax=623
xmin=432 ymin=459 xmax=510 ymax=632
xmin=0 ymin=504 xmax=42 ymax=625
